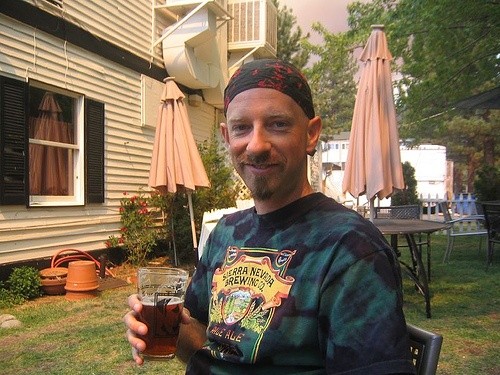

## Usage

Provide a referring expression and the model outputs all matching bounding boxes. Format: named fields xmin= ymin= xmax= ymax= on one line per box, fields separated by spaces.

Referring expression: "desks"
xmin=367 ymin=219 xmax=453 ymax=319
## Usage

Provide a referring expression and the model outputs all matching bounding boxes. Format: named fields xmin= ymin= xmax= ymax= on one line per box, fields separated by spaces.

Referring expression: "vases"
xmin=64 ymin=287 xmax=99 ymax=301
xmin=38 ymin=265 xmax=69 ymax=296
xmin=64 ymin=260 xmax=100 ymax=291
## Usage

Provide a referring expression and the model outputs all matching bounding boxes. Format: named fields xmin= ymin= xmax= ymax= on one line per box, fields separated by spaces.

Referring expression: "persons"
xmin=124 ymin=59 xmax=418 ymax=375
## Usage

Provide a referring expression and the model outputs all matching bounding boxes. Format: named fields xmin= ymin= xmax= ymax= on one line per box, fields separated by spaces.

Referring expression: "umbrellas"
xmin=341 ymin=24 xmax=406 ymax=225
xmin=29 ymin=91 xmax=74 ymax=196
xmin=148 ymin=77 xmax=211 ymax=265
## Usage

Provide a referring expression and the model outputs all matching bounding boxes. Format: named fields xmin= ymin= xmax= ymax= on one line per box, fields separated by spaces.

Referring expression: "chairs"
xmin=375 ymin=203 xmax=431 ymax=284
xmin=480 ymin=201 xmax=500 ymax=271
xmin=185 ymin=320 xmax=445 ymax=375
xmin=438 ymin=200 xmax=491 ymax=264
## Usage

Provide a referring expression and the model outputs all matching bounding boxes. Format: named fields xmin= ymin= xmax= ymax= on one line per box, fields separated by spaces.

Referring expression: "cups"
xmin=135 ymin=266 xmax=189 ymax=361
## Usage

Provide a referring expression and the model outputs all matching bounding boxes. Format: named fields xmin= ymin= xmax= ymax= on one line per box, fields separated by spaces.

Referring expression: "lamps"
xmin=188 ymin=94 xmax=203 ymax=107
